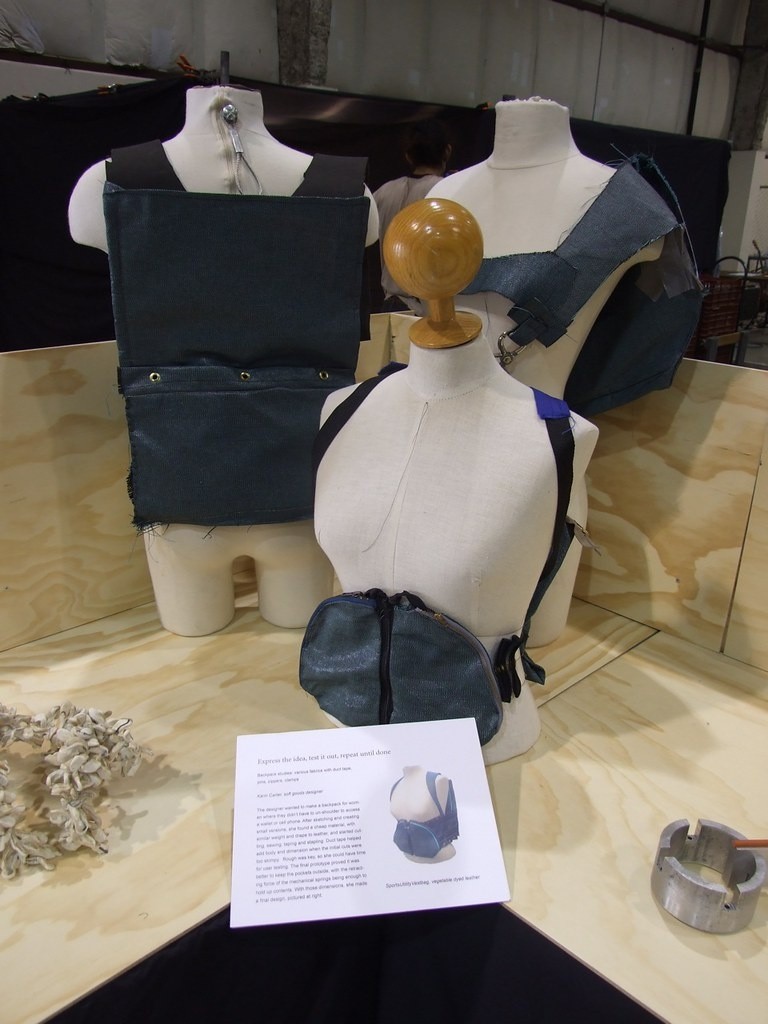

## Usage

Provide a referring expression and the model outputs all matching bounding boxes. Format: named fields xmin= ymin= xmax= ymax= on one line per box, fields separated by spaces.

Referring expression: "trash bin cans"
xmin=720 ymin=272 xmax=763 ymax=320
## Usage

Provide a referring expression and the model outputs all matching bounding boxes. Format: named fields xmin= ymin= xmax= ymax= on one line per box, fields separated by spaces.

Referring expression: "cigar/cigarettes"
xmin=733 ymin=840 xmax=768 ymax=851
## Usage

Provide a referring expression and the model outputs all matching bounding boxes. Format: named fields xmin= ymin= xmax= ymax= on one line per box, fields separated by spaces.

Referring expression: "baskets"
xmin=696 ymin=272 xmax=744 ymax=337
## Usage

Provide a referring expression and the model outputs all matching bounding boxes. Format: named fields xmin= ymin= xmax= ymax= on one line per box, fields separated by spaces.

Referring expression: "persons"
xmin=315 ymin=312 xmax=600 ymax=767
xmin=424 ymin=97 xmax=704 ymax=646
xmin=371 ymin=118 xmax=454 ymax=313
xmin=68 ymin=84 xmax=380 ymax=636
xmin=388 ymin=767 xmax=460 ymax=867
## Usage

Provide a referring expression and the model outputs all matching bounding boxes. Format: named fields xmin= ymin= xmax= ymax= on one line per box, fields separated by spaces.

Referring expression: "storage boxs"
xmin=699 ymin=274 xmax=742 ymax=363
